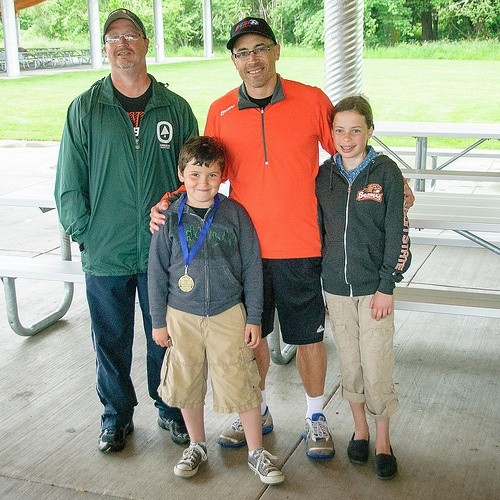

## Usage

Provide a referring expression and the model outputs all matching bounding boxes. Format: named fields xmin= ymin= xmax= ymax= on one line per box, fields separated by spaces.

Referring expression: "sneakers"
xmin=173 ymin=442 xmax=208 ymax=478
xmin=217 ymin=405 xmax=274 ymax=447
xmin=98 ymin=419 xmax=134 ymax=452
xmin=248 ymin=447 xmax=285 ymax=484
xmin=301 ymin=413 xmax=335 ymax=458
xmin=157 ymin=415 xmax=190 ymax=444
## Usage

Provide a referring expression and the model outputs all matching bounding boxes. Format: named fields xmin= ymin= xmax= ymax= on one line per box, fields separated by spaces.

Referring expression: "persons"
xmin=149 ymin=17 xmax=415 ymax=460
xmin=316 ymin=98 xmax=411 ymax=479
xmin=53 ymin=8 xmax=190 ymax=452
xmin=148 ymin=138 xmax=284 ymax=484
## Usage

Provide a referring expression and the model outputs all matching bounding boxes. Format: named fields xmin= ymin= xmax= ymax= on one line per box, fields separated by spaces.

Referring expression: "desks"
xmin=0 ymin=169 xmax=64 ymax=209
xmin=372 ymin=121 xmax=500 ymax=192
xmin=403 ymin=192 xmax=500 ymax=231
xmin=0 ymin=47 xmax=104 ymax=71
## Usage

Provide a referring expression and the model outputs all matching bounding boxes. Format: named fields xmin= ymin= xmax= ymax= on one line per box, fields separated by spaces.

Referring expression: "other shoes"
xmin=373 ymin=444 xmax=398 ymax=480
xmin=347 ymin=432 xmax=370 ymax=465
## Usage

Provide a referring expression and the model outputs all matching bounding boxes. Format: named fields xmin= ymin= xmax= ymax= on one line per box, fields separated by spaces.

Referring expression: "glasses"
xmin=232 ymin=43 xmax=275 ymax=61
xmin=104 ymin=33 xmax=145 ymax=44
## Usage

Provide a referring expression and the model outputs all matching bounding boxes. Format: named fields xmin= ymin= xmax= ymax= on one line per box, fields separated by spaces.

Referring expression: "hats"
xmin=103 ymin=8 xmax=146 ymax=38
xmin=227 ymin=17 xmax=277 ymax=50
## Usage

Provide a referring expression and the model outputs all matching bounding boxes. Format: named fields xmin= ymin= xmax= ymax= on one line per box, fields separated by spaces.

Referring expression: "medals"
xmin=178 ymin=275 xmax=194 ymax=292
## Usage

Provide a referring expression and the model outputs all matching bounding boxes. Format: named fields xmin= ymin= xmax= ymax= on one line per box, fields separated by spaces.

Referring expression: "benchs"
xmin=0 ymin=251 xmax=86 ymax=284
xmin=372 ymin=145 xmax=500 ymax=321
xmin=0 ymin=55 xmax=108 ymax=71
